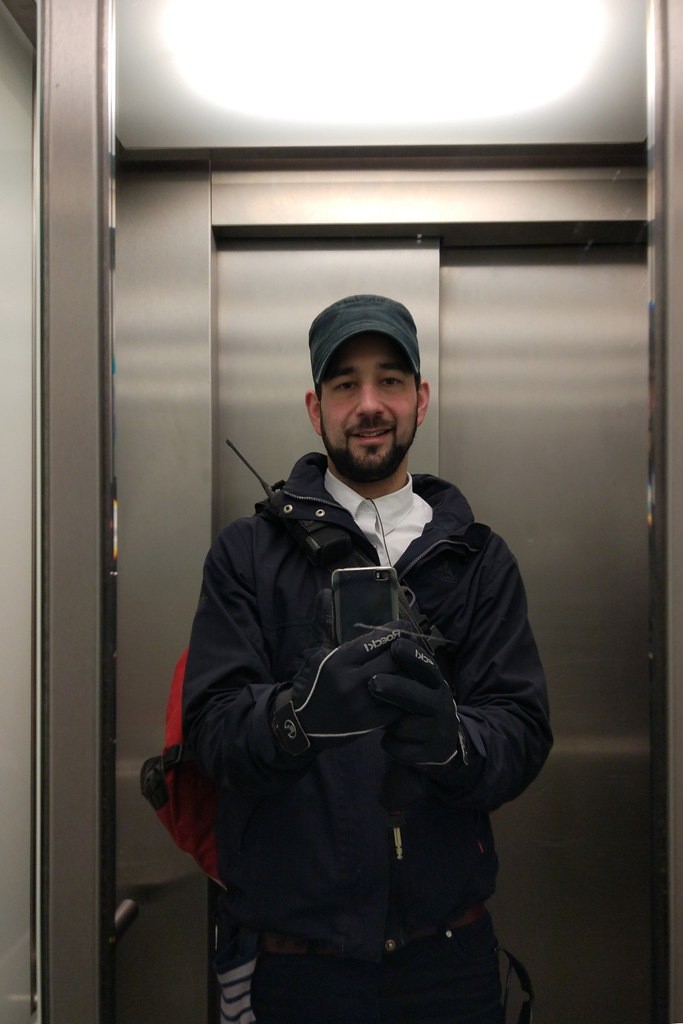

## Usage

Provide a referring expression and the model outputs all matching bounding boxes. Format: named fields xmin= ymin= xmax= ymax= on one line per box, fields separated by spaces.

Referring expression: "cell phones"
xmin=332 ymin=566 xmax=400 ymax=647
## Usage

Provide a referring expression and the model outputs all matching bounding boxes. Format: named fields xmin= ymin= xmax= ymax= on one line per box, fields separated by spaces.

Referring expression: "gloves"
xmin=367 ymin=638 xmax=487 ymax=769
xmin=273 ymin=619 xmax=416 ymax=757
xmin=212 ymin=935 xmax=257 ymax=1024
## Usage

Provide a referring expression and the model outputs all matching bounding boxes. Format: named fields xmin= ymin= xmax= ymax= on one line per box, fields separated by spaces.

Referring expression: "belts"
xmin=257 ymin=901 xmax=488 ymax=955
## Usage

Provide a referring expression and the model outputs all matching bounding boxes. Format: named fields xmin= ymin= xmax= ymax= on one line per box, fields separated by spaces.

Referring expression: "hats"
xmin=308 ymin=294 xmax=421 ymax=384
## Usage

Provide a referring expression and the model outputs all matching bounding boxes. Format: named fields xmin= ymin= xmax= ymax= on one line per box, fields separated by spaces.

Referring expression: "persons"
xmin=182 ymin=294 xmax=554 ymax=1024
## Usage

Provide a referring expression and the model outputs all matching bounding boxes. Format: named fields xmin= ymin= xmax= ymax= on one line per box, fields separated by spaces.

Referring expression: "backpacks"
xmin=136 ymin=637 xmax=229 ymax=885
